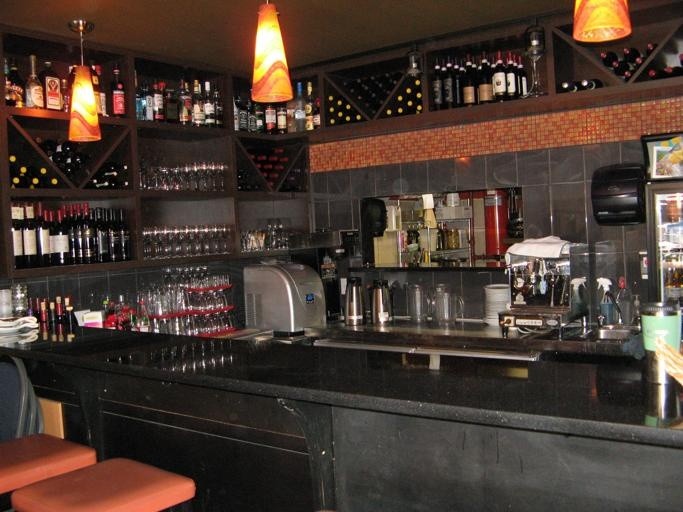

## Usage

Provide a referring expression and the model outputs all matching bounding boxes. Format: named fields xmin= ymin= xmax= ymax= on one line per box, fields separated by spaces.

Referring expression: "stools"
xmin=0 ymin=433 xmax=98 ymax=495
xmin=11 ymin=458 xmax=197 ymax=512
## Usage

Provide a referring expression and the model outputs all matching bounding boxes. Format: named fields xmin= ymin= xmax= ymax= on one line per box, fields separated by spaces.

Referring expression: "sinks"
xmin=578 ymin=325 xmax=636 ymax=341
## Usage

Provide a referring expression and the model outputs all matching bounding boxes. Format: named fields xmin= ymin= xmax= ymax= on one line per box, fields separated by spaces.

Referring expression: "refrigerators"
xmin=640 ymin=132 xmax=683 ymax=307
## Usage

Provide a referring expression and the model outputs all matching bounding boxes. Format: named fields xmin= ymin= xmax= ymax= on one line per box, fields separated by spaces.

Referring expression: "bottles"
xmin=233 ymin=81 xmax=321 ymax=134
xmin=142 ymin=224 xmax=234 ymax=259
xmin=328 ymin=73 xmax=423 ymax=125
xmin=11 ymin=201 xmax=130 ymax=269
xmin=432 ymin=50 xmax=527 ymax=111
xmin=10 ymin=136 xmax=130 ymax=190
xmin=135 ymin=70 xmax=224 ymax=128
xmin=237 ymin=149 xmax=307 ymax=191
xmin=27 ymin=266 xmax=234 ymax=338
xmin=104 ymin=340 xmax=235 ymax=374
xmin=241 ymin=223 xmax=294 ymax=252
xmin=5 ymin=55 xmax=126 ymax=117
xmin=139 ymin=162 xmax=227 ymax=192
xmin=562 ymin=44 xmax=683 ymax=93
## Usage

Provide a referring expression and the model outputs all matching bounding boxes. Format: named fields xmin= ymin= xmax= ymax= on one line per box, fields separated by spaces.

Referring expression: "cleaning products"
xmin=597 ymin=277 xmax=614 ymax=324
xmin=571 ymin=274 xmax=590 ymax=319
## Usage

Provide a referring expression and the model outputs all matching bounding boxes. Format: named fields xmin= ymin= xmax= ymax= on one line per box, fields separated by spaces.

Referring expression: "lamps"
xmin=572 ymin=0 xmax=632 ymax=43
xmin=252 ymin=0 xmax=295 ymax=103
xmin=67 ymin=19 xmax=103 ymax=141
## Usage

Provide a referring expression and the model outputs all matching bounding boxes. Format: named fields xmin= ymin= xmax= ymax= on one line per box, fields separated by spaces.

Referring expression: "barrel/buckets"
xmin=613 ymin=276 xmax=633 ymax=325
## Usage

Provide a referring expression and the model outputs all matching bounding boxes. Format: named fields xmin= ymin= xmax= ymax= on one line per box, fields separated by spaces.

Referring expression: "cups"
xmin=408 ymin=284 xmax=464 ymax=326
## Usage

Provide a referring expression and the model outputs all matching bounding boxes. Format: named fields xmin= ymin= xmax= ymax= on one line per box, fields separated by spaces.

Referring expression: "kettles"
xmin=346 ymin=278 xmax=392 ymax=327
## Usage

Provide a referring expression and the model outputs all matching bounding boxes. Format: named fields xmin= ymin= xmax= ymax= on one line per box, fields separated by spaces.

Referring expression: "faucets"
xmin=605 ymin=290 xmax=625 ymax=325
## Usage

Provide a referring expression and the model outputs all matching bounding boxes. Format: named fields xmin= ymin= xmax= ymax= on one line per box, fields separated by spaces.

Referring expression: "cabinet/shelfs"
xmin=276 ymin=0 xmax=683 ymax=145
xmin=0 ymin=25 xmax=315 ymax=281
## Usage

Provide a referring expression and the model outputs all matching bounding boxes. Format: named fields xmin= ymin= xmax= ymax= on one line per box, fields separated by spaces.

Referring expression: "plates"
xmin=483 ymin=284 xmax=511 ymax=327
